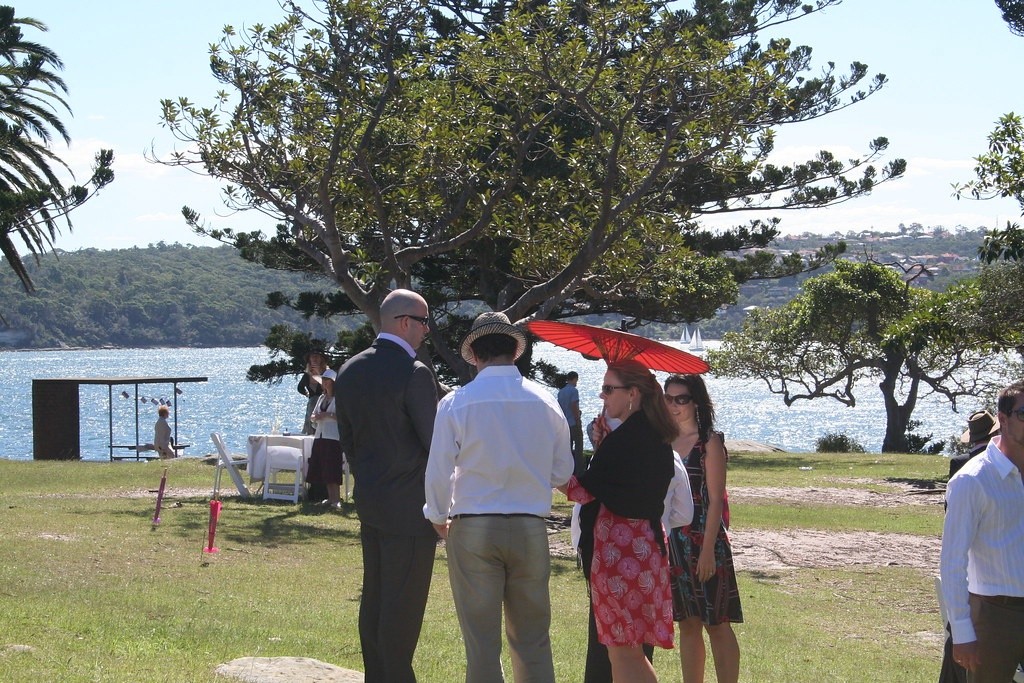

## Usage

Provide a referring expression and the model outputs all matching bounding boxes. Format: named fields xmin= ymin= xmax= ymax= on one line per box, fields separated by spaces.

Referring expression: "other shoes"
xmin=322 ymin=499 xmax=329 ymax=504
xmin=331 ymin=500 xmax=342 ymax=508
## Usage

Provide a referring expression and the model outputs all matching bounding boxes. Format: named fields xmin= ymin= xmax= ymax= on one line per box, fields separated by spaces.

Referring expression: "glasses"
xmin=601 ymin=384 xmax=631 ymax=396
xmin=394 ymin=313 xmax=429 ymax=326
xmin=1008 ymin=407 xmax=1024 ymax=421
xmin=664 ymin=393 xmax=693 ymax=405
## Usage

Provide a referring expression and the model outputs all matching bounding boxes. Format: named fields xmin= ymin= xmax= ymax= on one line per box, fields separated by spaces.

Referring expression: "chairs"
xmin=263 ymin=436 xmax=305 ymax=504
xmin=210 ymin=433 xmax=250 ymax=497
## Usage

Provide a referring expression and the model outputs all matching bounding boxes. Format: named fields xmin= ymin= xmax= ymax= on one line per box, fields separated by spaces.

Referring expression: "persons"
xmin=297 ymin=288 xmax=744 ymax=683
xmin=941 ymin=380 xmax=1024 ymax=683
xmin=155 ymin=407 xmax=176 ymax=458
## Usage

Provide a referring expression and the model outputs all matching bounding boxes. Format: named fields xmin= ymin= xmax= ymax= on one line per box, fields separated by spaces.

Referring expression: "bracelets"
xmin=330 ymin=412 xmax=333 ymax=417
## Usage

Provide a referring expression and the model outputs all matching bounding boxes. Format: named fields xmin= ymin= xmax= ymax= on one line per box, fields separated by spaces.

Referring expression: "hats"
xmin=459 ymin=312 xmax=527 ymax=366
xmin=961 ymin=410 xmax=999 ymax=442
xmin=311 ymin=369 xmax=337 ymax=385
xmin=304 ymin=347 xmax=326 ymax=363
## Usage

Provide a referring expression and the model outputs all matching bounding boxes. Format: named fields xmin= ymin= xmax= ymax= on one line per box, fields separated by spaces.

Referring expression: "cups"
xmin=282 ymin=430 xmax=291 ymax=436
xmin=306 ymin=363 xmax=314 ymax=375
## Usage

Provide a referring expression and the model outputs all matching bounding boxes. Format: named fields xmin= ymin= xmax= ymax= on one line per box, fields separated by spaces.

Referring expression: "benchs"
xmin=112 ymin=457 xmax=160 ymax=462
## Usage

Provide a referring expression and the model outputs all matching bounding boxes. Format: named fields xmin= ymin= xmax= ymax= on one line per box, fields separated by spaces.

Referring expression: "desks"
xmin=108 ymin=445 xmax=190 ymax=450
xmin=246 ymin=434 xmax=349 ymax=503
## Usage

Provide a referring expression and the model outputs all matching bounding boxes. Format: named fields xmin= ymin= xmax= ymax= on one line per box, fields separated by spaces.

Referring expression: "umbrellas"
xmin=528 ymin=319 xmax=710 ymax=456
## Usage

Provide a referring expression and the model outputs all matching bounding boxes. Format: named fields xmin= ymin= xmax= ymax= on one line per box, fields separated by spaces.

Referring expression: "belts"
xmin=448 ymin=513 xmax=545 ymax=518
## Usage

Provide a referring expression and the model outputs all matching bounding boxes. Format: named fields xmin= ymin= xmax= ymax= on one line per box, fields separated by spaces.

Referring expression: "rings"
xmin=709 ymin=571 xmax=713 ymax=575
xmin=958 ymin=659 xmax=962 ymax=663
